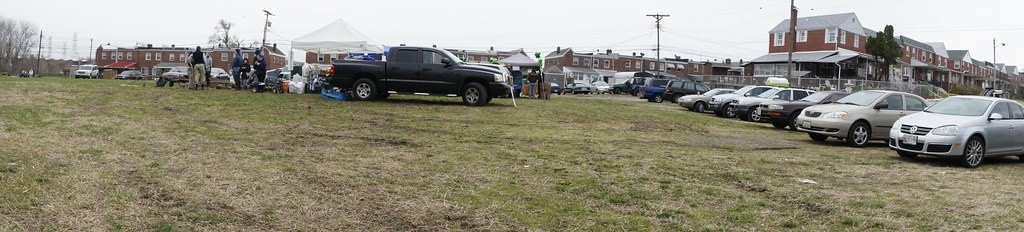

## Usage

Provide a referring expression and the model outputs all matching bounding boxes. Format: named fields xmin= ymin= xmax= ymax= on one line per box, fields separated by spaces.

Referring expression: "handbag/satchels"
xmin=233 ymin=66 xmax=239 ymax=75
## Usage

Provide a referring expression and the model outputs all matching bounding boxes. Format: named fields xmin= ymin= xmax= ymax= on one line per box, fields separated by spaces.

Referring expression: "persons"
xmin=232 ymin=49 xmax=243 ymax=90
xmin=253 ymin=50 xmax=267 ymax=83
xmin=240 ymin=58 xmax=251 ymax=81
xmin=538 ymin=72 xmax=544 ymax=98
xmin=187 ymin=46 xmax=212 ymax=90
xmin=528 ymin=69 xmax=538 ymax=98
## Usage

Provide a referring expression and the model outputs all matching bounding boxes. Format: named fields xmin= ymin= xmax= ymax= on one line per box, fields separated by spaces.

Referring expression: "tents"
xmin=290 ymin=19 xmax=384 ymax=67
xmin=498 ymin=53 xmax=545 ymax=98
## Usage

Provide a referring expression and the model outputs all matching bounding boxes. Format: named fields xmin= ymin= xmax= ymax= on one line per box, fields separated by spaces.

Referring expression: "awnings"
xmin=105 ymin=62 xmax=138 ymax=69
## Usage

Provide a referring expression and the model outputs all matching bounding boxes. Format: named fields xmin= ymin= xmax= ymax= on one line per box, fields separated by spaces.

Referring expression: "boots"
xmin=201 ymin=84 xmax=204 ymax=90
xmin=194 ymin=84 xmax=198 ymax=90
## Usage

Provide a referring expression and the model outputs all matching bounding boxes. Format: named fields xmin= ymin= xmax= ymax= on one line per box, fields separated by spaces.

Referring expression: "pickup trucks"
xmin=325 ymin=46 xmax=512 ymax=107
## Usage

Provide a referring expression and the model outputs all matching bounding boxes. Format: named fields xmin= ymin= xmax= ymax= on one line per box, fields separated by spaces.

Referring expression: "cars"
xmin=796 ymin=90 xmax=933 ymax=147
xmin=464 ymin=62 xmax=514 ymax=92
xmin=756 ymin=90 xmax=850 ymax=132
xmin=662 ymin=79 xmax=712 ymax=104
xmin=727 ymin=88 xmax=788 ymax=123
xmin=562 ymin=83 xmax=591 ymax=95
xmin=639 ymin=78 xmax=670 ymax=103
xmin=74 ymin=65 xmax=99 ymax=79
xmin=589 ymin=82 xmax=610 ymax=94
xmin=210 ymin=68 xmax=230 ymax=81
xmin=709 ymin=85 xmax=779 ymax=118
xmin=677 ymin=88 xmax=737 ymax=113
xmin=629 ymin=77 xmax=641 ymax=96
xmin=889 ymin=96 xmax=1024 ymax=168
xmin=551 ymin=83 xmax=562 ymax=95
xmin=230 ymin=66 xmax=256 ymax=88
xmin=114 ymin=70 xmax=144 ymax=80
xmin=162 ymin=67 xmax=190 ymax=83
xmin=609 ymin=79 xmax=634 ymax=94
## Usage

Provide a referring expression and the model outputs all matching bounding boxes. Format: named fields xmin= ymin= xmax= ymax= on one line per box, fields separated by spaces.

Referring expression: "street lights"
xmin=994 ymin=43 xmax=1005 ymax=89
